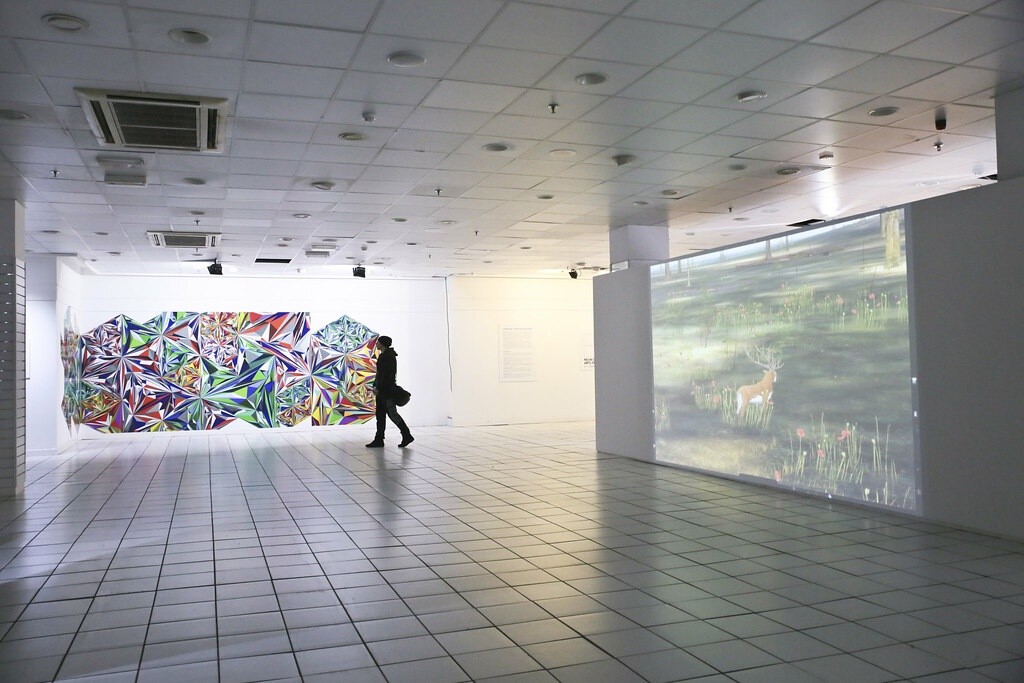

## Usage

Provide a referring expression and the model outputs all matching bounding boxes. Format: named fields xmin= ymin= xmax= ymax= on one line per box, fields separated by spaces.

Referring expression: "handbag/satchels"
xmin=393 ymin=384 xmax=411 ymax=406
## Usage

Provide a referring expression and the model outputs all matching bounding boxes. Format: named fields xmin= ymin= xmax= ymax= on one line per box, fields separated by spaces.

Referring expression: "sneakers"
xmin=398 ymin=436 xmax=414 ymax=447
xmin=366 ymin=440 xmax=385 ymax=447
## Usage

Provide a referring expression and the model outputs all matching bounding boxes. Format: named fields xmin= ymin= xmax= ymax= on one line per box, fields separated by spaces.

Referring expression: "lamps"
xmin=734 ymin=90 xmax=768 ymax=103
xmin=311 ymin=181 xmax=335 ymax=190
xmin=386 ymin=51 xmax=426 ymax=68
xmin=575 ymin=73 xmax=608 ymax=86
xmin=612 ymin=155 xmax=636 ymax=165
xmin=353 ymin=264 xmax=366 ymax=278
xmin=207 ymin=259 xmax=223 ymax=275
xmin=569 ymin=269 xmax=577 ymax=278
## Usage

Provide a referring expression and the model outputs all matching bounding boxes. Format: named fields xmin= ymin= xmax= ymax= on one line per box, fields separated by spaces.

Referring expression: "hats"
xmin=378 ymin=336 xmax=392 ymax=348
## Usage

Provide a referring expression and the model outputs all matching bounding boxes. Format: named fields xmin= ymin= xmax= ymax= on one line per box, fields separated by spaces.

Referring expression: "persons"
xmin=365 ymin=336 xmax=414 ymax=447
